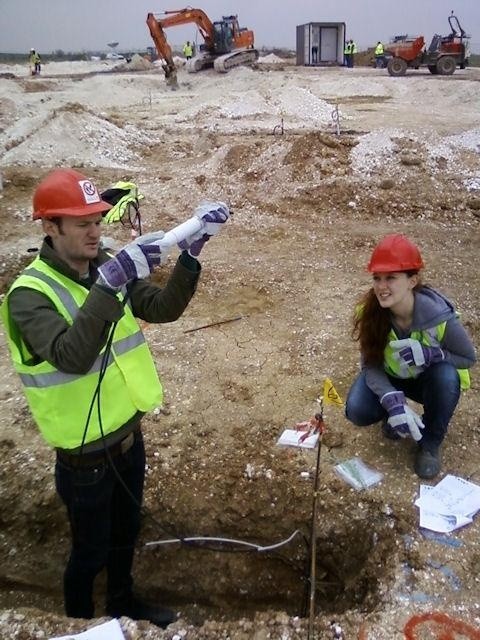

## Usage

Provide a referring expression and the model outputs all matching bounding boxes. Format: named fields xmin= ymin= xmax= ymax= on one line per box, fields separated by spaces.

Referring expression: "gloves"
xmin=97 ymin=231 xmax=173 ymax=292
xmin=380 ymin=390 xmax=425 ymax=441
xmin=388 ymin=338 xmax=445 ymax=368
xmin=178 ymin=201 xmax=231 ymax=259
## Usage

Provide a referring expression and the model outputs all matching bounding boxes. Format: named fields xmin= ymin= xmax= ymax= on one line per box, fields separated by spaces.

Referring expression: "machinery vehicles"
xmin=377 ymin=13 xmax=469 ymax=75
xmin=144 ymin=8 xmax=261 ymax=87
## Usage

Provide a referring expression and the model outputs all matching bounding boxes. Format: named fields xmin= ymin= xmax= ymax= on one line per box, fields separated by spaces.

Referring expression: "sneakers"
xmin=104 ymin=601 xmax=175 ymax=625
xmin=414 ymin=441 xmax=440 ymax=480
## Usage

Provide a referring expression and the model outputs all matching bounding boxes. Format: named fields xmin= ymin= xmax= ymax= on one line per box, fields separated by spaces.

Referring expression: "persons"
xmin=28 ymin=48 xmax=42 ymax=74
xmin=374 ymin=41 xmax=384 ymax=68
xmin=0 ymin=170 xmax=235 ymax=628
xmin=183 ymin=41 xmax=193 ymax=60
xmin=346 ymin=234 xmax=477 ymax=481
xmin=344 ymin=40 xmax=352 ymax=65
xmin=350 ymin=39 xmax=358 ymax=67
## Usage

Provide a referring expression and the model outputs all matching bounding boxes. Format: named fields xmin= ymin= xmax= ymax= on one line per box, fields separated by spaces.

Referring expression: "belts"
xmin=72 ymin=432 xmax=136 ymax=466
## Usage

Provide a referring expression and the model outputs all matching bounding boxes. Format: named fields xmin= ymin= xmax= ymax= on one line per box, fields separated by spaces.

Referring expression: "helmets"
xmin=366 ymin=234 xmax=424 ymax=272
xmin=31 ymin=169 xmax=115 ymax=218
